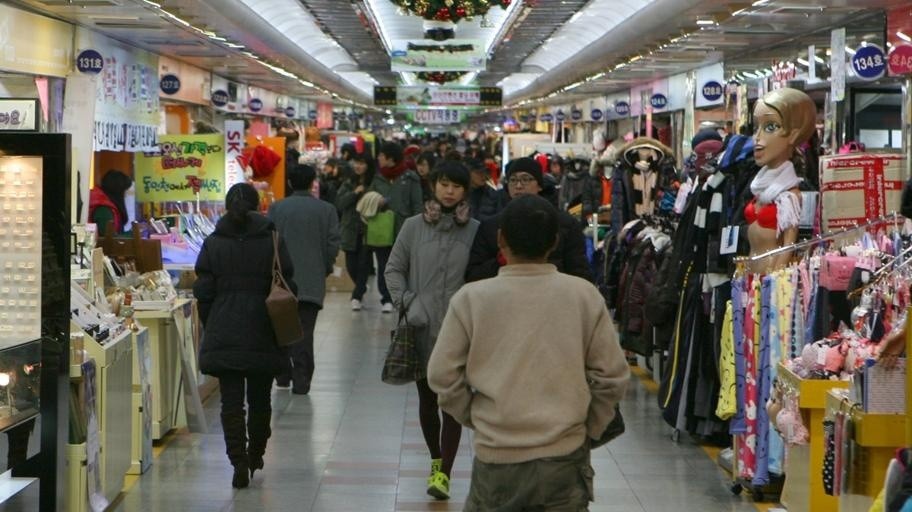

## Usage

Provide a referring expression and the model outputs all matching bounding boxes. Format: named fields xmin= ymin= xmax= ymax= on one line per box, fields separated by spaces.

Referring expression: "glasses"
xmin=508 ymin=174 xmax=535 ymax=185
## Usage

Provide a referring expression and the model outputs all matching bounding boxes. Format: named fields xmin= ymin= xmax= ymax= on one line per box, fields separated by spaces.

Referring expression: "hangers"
xmin=629 ymin=214 xmax=675 ymax=249
xmin=743 ymin=208 xmax=903 ymax=272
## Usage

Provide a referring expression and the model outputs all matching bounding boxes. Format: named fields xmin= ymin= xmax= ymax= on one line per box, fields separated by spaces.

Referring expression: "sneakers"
xmin=382 ymin=303 xmax=393 ymax=313
xmin=428 ymin=459 xmax=451 ymax=499
xmin=352 ymin=299 xmax=361 ymax=310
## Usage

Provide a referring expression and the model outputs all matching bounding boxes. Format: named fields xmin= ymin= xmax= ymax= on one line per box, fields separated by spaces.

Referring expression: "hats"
xmin=692 ymin=130 xmax=723 ymax=173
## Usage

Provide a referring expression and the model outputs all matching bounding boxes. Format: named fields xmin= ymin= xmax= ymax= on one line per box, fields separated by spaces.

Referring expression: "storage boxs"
xmin=812 ymin=148 xmax=908 ymax=247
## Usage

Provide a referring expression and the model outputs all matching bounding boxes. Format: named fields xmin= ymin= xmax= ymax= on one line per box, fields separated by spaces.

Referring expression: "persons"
xmin=88 ymin=168 xmax=133 ymax=237
xmin=383 ymin=159 xmax=482 ymax=503
xmin=743 ymin=87 xmax=817 ymax=272
xmin=192 ymin=181 xmax=295 ymax=489
xmin=426 ymin=193 xmax=633 ymax=512
xmin=266 ymin=164 xmax=339 ymax=394
xmin=320 ymin=125 xmax=561 ymax=313
xmin=609 ymin=134 xmax=675 ymax=237
xmin=467 ymin=155 xmax=590 ymax=284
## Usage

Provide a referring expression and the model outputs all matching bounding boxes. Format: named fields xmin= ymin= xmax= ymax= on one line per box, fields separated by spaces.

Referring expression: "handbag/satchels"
xmin=382 ymin=288 xmax=426 ymax=385
xmin=362 ymin=210 xmax=396 ymax=247
xmin=265 ymin=270 xmax=305 ymax=345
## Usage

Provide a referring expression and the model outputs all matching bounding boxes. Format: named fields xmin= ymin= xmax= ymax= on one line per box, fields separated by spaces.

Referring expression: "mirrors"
xmin=0 ymin=338 xmax=41 ymax=434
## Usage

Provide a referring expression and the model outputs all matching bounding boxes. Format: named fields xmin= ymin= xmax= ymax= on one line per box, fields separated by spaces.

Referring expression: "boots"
xmin=220 ymin=407 xmax=271 ymax=487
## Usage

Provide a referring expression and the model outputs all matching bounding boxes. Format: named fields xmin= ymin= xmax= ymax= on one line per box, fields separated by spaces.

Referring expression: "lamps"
xmin=22 ymin=361 xmax=40 ymax=374
